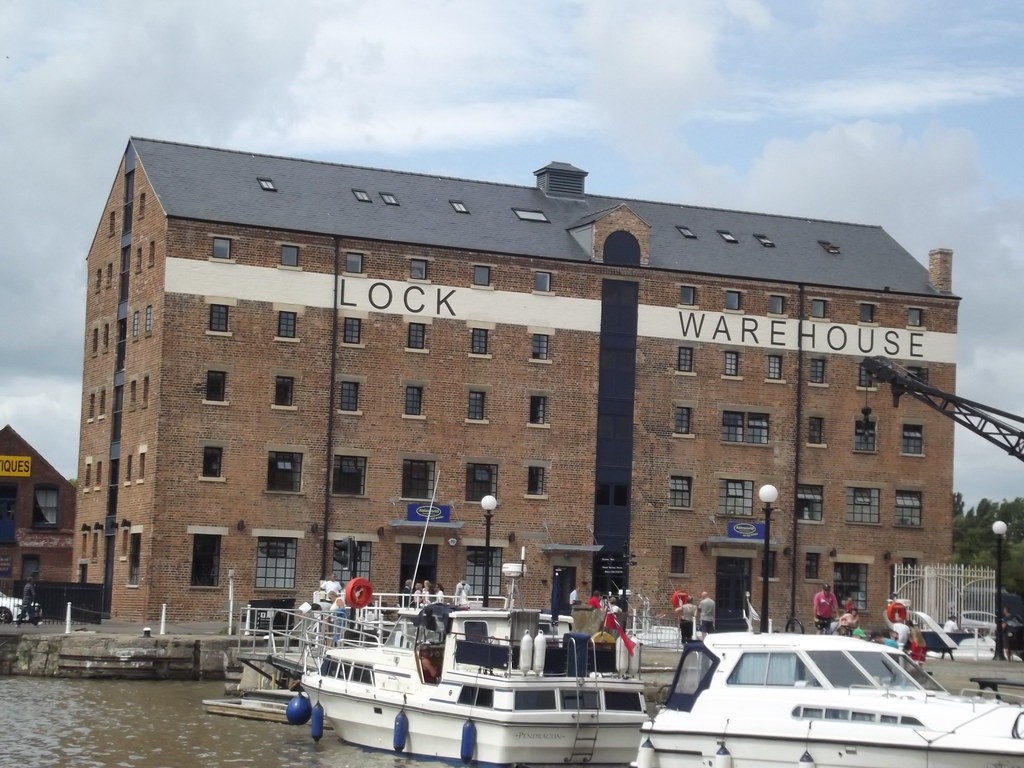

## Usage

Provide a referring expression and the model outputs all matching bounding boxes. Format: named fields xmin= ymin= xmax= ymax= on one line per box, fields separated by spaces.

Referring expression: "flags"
xmin=604 ymin=598 xmax=636 ymax=656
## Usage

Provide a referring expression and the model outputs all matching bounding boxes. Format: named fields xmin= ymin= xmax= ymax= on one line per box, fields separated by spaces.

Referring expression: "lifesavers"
xmin=671 ymin=589 xmax=688 ymax=608
xmin=888 ymin=601 xmax=907 ymax=623
xmin=593 ymin=631 xmax=616 ymax=644
xmin=346 ymin=576 xmax=373 ymax=609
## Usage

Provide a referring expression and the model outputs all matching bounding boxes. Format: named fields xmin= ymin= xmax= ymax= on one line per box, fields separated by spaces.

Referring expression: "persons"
xmin=1002 ymin=605 xmax=1024 ymax=662
xmin=569 ymin=585 xmax=580 ymax=615
xmin=867 ymin=629 xmax=899 ymax=683
xmin=674 ymin=595 xmax=698 ymax=649
xmin=398 ymin=579 xmax=445 ymax=619
xmin=586 ymin=590 xmax=622 ymax=634
xmin=15 ymin=577 xmax=44 ymax=628
xmin=813 ymin=583 xmax=859 ymax=637
xmin=453 ymin=574 xmax=473 ymax=606
xmin=698 ymin=591 xmax=715 ymax=640
xmin=943 ymin=615 xmax=965 ymax=633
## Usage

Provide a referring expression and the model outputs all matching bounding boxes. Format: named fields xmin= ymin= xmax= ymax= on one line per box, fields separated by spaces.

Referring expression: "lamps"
xmin=830 ymin=548 xmax=837 ymax=558
xmin=377 ymin=527 xmax=384 ymax=536
xmin=120 ymin=519 xmax=131 ymax=528
xmin=237 ymin=519 xmax=245 ymax=531
xmin=93 ymin=522 xmax=104 ymax=530
xmin=81 ymin=523 xmax=91 ymax=532
xmin=508 ymin=533 xmax=515 ymax=543
xmin=884 ymin=552 xmax=890 ymax=563
xmin=109 ymin=520 xmax=118 ymax=528
xmin=784 ymin=547 xmax=791 ymax=556
xmin=311 ymin=523 xmax=319 ymax=534
xmin=699 ymin=542 xmax=707 ymax=552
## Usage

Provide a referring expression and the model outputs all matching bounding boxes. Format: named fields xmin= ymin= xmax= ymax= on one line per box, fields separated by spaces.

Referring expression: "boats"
xmin=625 ymin=620 xmax=1023 ymax=768
xmin=201 ymin=690 xmax=332 ymax=730
xmin=281 ymin=466 xmax=650 ymax=766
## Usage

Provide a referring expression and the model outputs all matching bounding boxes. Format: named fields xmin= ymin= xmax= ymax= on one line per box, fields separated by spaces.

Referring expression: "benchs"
xmin=969 ymin=676 xmax=1024 ymax=699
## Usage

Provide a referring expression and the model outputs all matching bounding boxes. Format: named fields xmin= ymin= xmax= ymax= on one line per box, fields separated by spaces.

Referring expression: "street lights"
xmin=757 ymin=483 xmax=780 ymax=636
xmin=480 ymin=493 xmax=497 ymax=608
xmin=991 ymin=519 xmax=1007 ymax=661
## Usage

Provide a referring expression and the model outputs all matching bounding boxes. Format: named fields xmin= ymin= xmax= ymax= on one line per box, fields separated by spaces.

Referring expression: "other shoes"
xmin=34 ymin=625 xmax=39 ymax=627
xmin=15 ymin=624 xmax=20 ymax=628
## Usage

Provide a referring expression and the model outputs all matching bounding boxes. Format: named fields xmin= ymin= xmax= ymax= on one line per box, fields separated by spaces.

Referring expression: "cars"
xmin=961 ymin=610 xmax=1005 ymax=634
xmin=0 ymin=590 xmax=42 ymax=624
